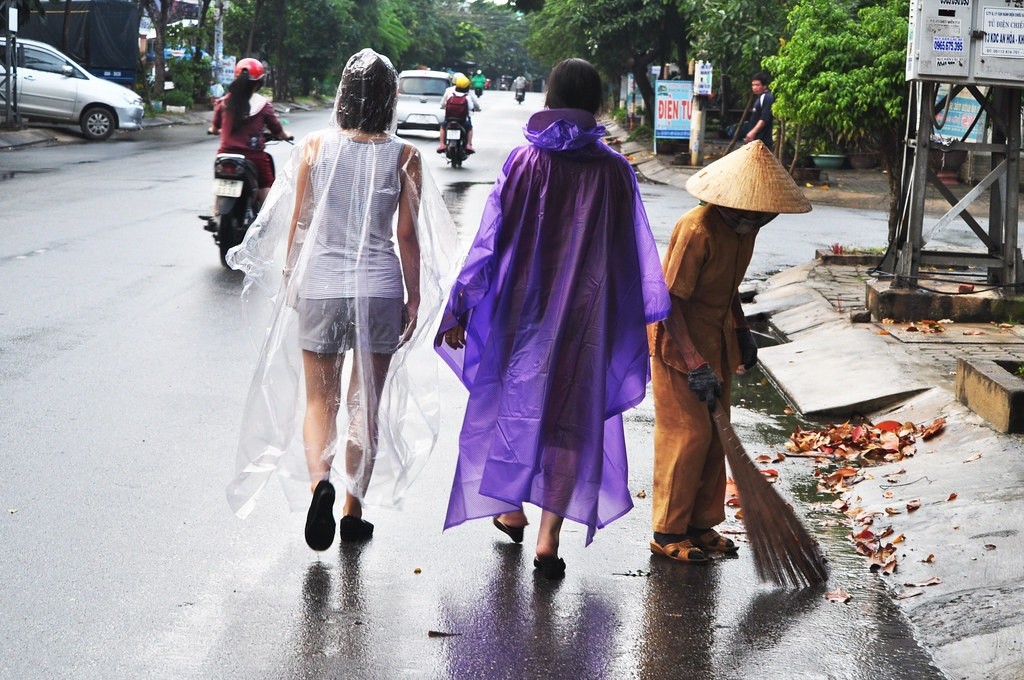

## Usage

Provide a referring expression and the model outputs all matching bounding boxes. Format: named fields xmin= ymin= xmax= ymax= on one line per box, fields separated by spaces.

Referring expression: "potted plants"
xmin=809 ymin=140 xmax=845 ymax=165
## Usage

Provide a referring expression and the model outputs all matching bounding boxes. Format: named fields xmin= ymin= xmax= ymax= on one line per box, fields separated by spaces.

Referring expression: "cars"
xmin=0 ymin=37 xmax=146 ymax=141
xmin=395 ymin=70 xmax=454 ymax=137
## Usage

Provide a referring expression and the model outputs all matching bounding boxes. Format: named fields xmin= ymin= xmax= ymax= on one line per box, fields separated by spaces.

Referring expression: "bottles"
xmin=251 ymin=136 xmax=259 ymax=147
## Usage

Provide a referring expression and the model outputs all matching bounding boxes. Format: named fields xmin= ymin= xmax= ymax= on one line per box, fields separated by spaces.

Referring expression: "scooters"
xmin=440 ymin=106 xmax=481 ymax=168
xmin=198 ymin=131 xmax=294 ymax=264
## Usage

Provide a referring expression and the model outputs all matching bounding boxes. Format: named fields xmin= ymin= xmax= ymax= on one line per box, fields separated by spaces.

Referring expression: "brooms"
xmin=706 ymin=393 xmax=832 ymax=590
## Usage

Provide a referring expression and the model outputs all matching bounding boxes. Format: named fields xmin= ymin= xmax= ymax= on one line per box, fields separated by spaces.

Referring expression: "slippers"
xmin=650 ymin=539 xmax=709 ymax=563
xmin=690 ymin=530 xmax=740 ymax=552
xmin=492 ymin=514 xmax=530 ymax=543
xmin=534 ymin=555 xmax=567 ymax=571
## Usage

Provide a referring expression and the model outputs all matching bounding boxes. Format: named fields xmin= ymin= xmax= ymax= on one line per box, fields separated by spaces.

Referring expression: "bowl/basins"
xmin=811 ymin=154 xmax=847 ymax=167
xmin=848 ymin=152 xmax=879 ymax=168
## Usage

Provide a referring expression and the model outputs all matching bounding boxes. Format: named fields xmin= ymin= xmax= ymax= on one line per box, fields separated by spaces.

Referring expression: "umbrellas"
xmin=146 ymin=45 xmax=209 ymax=61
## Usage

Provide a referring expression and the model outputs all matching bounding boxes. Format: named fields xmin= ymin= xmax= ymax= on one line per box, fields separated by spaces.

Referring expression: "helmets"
xmin=232 ymin=58 xmax=267 ymax=81
xmin=452 ymin=73 xmax=466 ymax=84
xmin=456 ymin=77 xmax=470 ymax=88
xmin=477 ymin=70 xmax=481 ymax=74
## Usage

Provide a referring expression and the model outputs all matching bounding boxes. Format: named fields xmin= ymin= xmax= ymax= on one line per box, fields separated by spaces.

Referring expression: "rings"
xmin=445 ymin=336 xmax=450 ymax=338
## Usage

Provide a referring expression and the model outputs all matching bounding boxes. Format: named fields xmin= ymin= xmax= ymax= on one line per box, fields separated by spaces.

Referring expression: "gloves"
xmin=687 ymin=365 xmax=725 ymax=414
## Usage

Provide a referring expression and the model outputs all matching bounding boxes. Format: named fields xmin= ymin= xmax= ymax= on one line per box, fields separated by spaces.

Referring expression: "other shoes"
xmin=465 ymin=145 xmax=476 ymax=154
xmin=304 ymin=479 xmax=336 ymax=552
xmin=340 ymin=514 xmax=374 ymax=539
xmin=437 ymin=145 xmax=447 ymax=153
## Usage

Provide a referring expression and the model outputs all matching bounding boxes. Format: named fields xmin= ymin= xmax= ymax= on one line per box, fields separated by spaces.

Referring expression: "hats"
xmin=684 ymin=137 xmax=813 ymax=216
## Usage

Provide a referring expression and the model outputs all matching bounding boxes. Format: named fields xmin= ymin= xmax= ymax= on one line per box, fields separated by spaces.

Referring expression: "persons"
xmin=647 ymin=138 xmax=813 ymax=561
xmin=434 ymin=60 xmax=670 ymax=571
xmin=742 ymin=72 xmax=775 ymax=149
xmin=435 ymin=71 xmax=480 ymax=152
xmin=471 ymin=70 xmax=486 ymax=89
xmin=513 ymin=73 xmax=528 ymax=96
xmin=209 ymin=56 xmax=287 ymax=203
xmin=227 ymin=48 xmax=461 ymax=549
